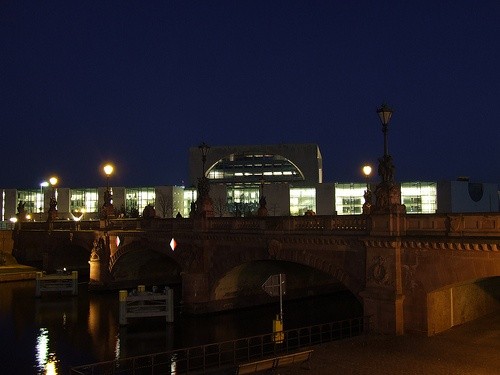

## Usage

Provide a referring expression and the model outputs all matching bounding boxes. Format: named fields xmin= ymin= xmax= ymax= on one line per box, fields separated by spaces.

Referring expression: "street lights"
xmin=47 ymin=175 xmax=61 ymax=221
xmin=258 ymin=174 xmax=269 ymax=217
xmin=196 ymin=142 xmax=214 ymax=218
xmin=374 ymin=102 xmax=403 ymax=213
xmin=102 ymin=161 xmax=116 ymax=218
xmin=362 ymin=161 xmax=373 ymax=213
